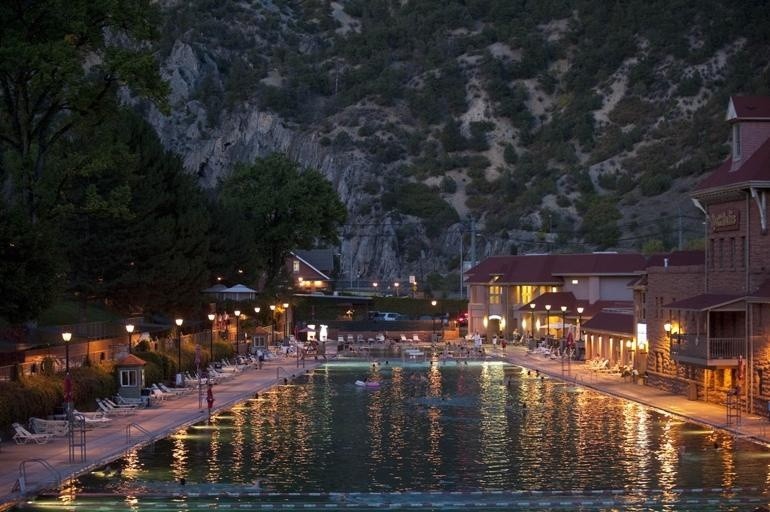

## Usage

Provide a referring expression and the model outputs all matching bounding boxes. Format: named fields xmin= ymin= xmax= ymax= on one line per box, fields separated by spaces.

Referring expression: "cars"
xmin=364 ymin=309 xmax=468 ymax=328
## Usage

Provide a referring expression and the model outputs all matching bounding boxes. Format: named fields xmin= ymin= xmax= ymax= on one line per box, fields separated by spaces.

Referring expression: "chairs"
xmin=337 ymin=333 xmax=421 ymax=345
xmin=13 ymin=346 xmax=296 ymax=445
xmin=526 ymin=343 xmax=622 ymax=373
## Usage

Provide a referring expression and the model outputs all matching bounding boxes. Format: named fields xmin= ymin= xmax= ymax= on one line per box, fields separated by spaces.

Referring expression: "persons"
xmin=373 ymin=360 xmax=388 ymax=371
xmin=507 ymin=368 xmax=545 ymax=408
xmin=256 ymin=349 xmax=263 ymax=362
xmin=431 ymin=359 xmax=468 ymax=365
xmin=409 ymin=372 xmax=417 ymax=381
xmin=473 ymin=330 xmax=530 ymax=354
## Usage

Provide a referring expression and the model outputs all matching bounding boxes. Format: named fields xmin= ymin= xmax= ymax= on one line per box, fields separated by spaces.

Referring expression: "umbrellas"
xmin=736 ymin=355 xmax=745 ymax=392
xmin=194 ymin=343 xmax=201 ymax=379
xmin=566 ymin=325 xmax=573 ymax=357
xmin=207 ymin=385 xmax=213 ymax=409
xmin=62 ymin=372 xmax=74 ymax=420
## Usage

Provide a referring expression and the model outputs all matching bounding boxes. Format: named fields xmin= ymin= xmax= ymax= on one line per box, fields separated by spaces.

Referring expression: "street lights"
xmin=372 ymin=282 xmax=377 ymax=295
xmin=431 ymin=299 xmax=438 ymax=341
xmin=176 ymin=318 xmax=184 ymax=388
xmin=530 ymin=303 xmax=584 ymax=352
xmin=412 ymin=281 xmax=417 ymax=298
xmin=208 ymin=303 xmax=290 ymax=362
xmin=125 ymin=324 xmax=135 ymax=353
xmin=395 ymin=282 xmax=400 ymax=296
xmin=337 ymin=252 xmax=345 ymax=296
xmin=356 ymin=272 xmax=361 ymax=296
xmin=62 ymin=332 xmax=72 ymax=371
